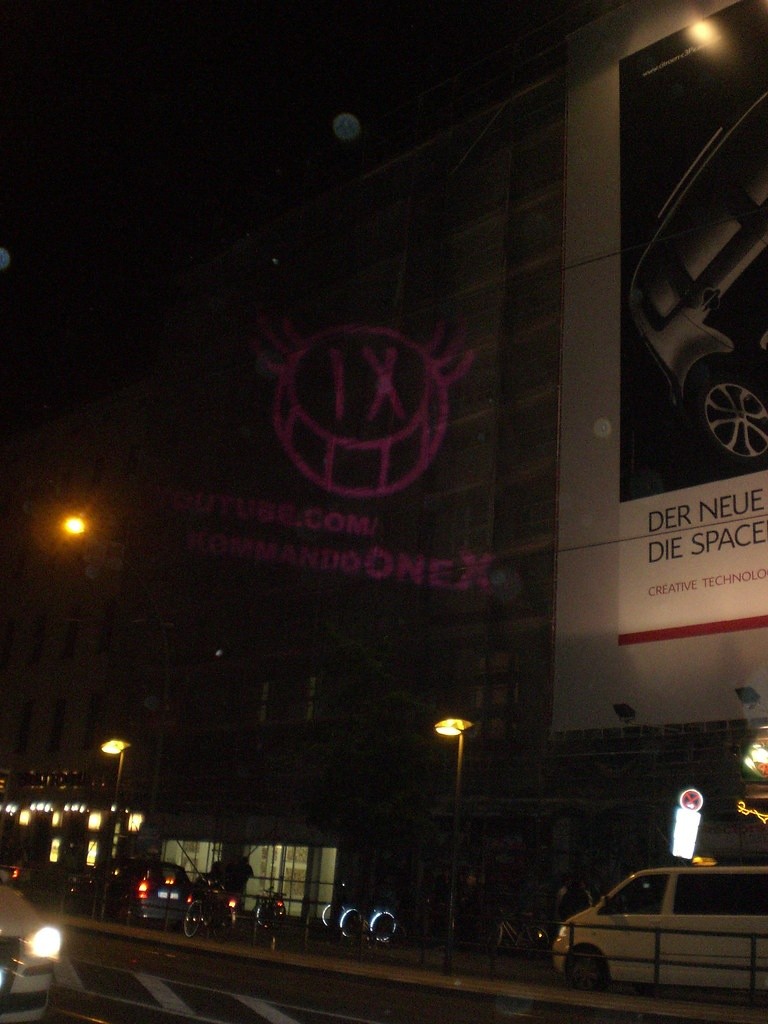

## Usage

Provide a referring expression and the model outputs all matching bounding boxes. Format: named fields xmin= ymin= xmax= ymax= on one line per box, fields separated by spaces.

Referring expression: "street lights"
xmin=98 ymin=739 xmax=132 ymax=922
xmin=433 ymin=718 xmax=471 ymax=977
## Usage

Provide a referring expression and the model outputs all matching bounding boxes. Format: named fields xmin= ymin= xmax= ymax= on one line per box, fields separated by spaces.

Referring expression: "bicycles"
xmin=255 ymin=885 xmax=287 ymax=928
xmin=484 ymin=907 xmax=549 ymax=955
xmin=184 ymin=879 xmax=238 ymax=944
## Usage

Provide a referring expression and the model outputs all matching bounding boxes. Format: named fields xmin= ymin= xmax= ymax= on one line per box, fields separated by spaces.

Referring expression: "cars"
xmin=0 ymin=874 xmax=65 ymax=1024
xmin=68 ymin=854 xmax=193 ymax=928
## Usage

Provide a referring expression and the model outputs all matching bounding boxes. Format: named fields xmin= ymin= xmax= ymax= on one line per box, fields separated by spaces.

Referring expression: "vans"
xmin=548 ymin=864 xmax=768 ymax=996
